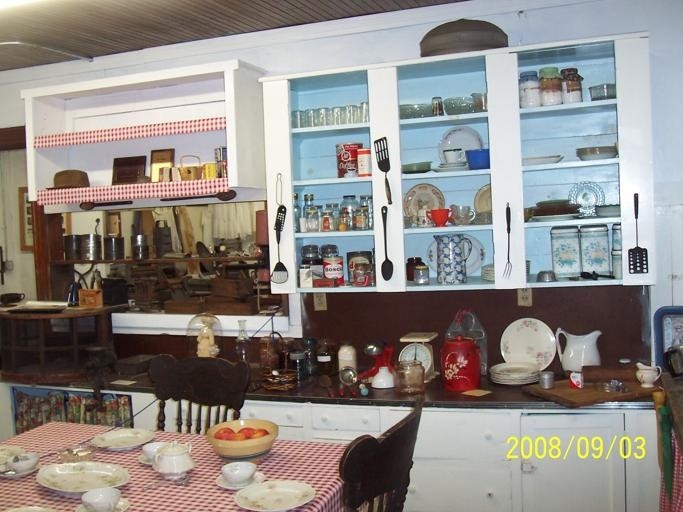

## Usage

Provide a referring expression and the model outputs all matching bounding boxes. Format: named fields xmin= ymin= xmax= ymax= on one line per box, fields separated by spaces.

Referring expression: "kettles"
xmin=67 ymin=282 xmax=81 ymax=306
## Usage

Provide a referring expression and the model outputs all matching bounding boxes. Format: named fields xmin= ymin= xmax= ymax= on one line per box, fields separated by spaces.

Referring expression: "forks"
xmin=502 ymin=202 xmax=513 ymax=281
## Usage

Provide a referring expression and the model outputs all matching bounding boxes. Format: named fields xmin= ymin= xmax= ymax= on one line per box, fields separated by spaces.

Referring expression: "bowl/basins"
xmin=537 ymin=200 xmax=571 ymax=207
xmin=595 ymin=204 xmax=620 ymax=218
xmin=221 ymin=461 xmax=256 ymax=484
xmin=535 ymin=271 xmax=557 ymax=284
xmin=443 ymin=96 xmax=474 ymax=115
xmin=142 ymin=441 xmax=169 ymax=460
xmin=400 ymin=104 xmax=431 ymax=118
xmin=465 ymin=149 xmax=489 ymax=169
xmin=207 ymin=418 xmax=279 ymax=459
xmin=7 ymin=453 xmax=39 ymax=473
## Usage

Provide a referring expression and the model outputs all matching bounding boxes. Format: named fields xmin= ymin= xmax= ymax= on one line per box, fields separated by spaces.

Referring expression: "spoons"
xmin=269 ymin=205 xmax=288 ymax=283
xmin=315 ymin=374 xmax=336 ymax=398
xmin=381 ymin=206 xmax=394 ymax=281
xmin=339 ymin=365 xmax=379 ymax=385
xmin=364 ymin=343 xmax=383 ymax=367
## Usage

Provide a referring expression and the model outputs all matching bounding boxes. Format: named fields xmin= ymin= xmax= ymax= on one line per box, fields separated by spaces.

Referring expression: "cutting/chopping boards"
xmin=520 ymin=378 xmax=662 ymax=407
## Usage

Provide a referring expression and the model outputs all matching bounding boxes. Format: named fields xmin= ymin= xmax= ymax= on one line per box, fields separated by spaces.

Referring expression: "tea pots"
xmin=152 ymin=442 xmax=196 ymax=480
xmin=556 ymin=327 xmax=602 ymax=370
xmin=636 ymin=362 xmax=662 ymax=387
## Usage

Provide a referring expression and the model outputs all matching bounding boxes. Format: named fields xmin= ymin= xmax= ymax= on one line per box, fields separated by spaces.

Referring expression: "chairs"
xmin=335 ymin=407 xmax=432 ymax=512
xmin=146 ymin=351 xmax=250 ymax=432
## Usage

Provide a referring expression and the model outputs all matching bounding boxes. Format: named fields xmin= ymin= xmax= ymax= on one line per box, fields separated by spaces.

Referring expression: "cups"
xmin=450 ymin=204 xmax=476 ymax=226
xmin=292 ymin=110 xmax=305 ymax=128
xmin=305 ymin=108 xmax=315 ymax=127
xmin=443 ymin=149 xmax=465 ymax=162
xmin=316 ymin=107 xmax=331 ymax=126
xmin=81 ymin=487 xmax=120 ymax=511
xmin=331 ymin=106 xmax=346 ymax=125
xmin=0 ymin=293 xmax=25 ymax=303
xmin=360 ymin=101 xmax=369 ymax=124
xmin=346 ymin=104 xmax=358 ymax=124
xmin=426 ymin=209 xmax=451 ymax=227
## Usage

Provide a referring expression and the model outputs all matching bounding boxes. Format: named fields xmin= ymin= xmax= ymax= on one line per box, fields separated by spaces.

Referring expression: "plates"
xmin=490 ymin=362 xmax=539 ymax=385
xmin=401 ymin=183 xmax=447 ymax=226
xmin=0 ymin=304 xmax=18 ymax=307
xmin=435 ymin=162 xmax=468 ymax=172
xmin=427 ymin=233 xmax=485 ymax=276
xmin=0 ymin=463 xmax=39 ymax=480
xmin=521 ymin=155 xmax=566 ymax=165
xmin=138 ymin=454 xmax=153 ymax=466
xmin=531 ymin=213 xmax=581 ymax=222
xmin=215 ymin=472 xmax=265 ymax=490
xmin=528 ymin=204 xmax=582 ymax=214
xmin=75 ymin=498 xmax=129 ymax=512
xmin=474 ymin=185 xmax=491 ymax=213
xmin=233 ymin=480 xmax=316 ymax=512
xmin=439 ymin=125 xmax=483 ymax=164
xmin=481 ymin=265 xmax=494 ymax=282
xmin=574 ymin=146 xmax=617 ymax=162
xmin=6 ymin=507 xmax=52 ymax=512
xmin=567 ymin=180 xmax=605 ymax=217
xmin=499 ymin=317 xmax=557 ymax=373
xmin=36 ymin=461 xmax=130 ymax=498
xmin=402 ymin=162 xmax=432 ymax=174
xmin=91 ymin=429 xmax=155 ymax=452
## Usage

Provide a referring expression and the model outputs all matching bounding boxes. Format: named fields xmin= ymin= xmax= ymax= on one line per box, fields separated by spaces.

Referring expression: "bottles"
xmin=259 ymin=337 xmax=279 ymax=372
xmin=519 ymin=71 xmax=541 ymax=109
xmin=339 ymin=207 xmax=346 ymax=231
xmin=353 ymin=263 xmax=371 ymax=286
xmin=431 ymin=96 xmax=444 ymax=116
xmin=332 ymin=203 xmax=339 ymax=229
xmin=317 ymin=206 xmax=323 ymax=230
xmin=326 ymin=203 xmax=331 ymax=210
xmin=338 ymin=341 xmax=356 ymax=372
xmin=414 ymin=266 xmax=428 ymax=285
xmin=292 ymin=194 xmax=300 ymax=232
xmin=345 ymin=211 xmax=350 ymax=226
xmin=352 ymin=208 xmax=368 ymax=231
xmin=341 ymin=195 xmax=359 ymax=230
xmin=306 ymin=209 xmax=319 ymax=232
xmin=561 ymin=68 xmax=584 ymax=105
xmin=303 ymin=194 xmax=313 ymax=218
xmin=539 ymin=67 xmax=562 ymax=106
xmin=323 ymin=257 xmax=344 ymax=286
xmin=360 ymin=195 xmax=374 ymax=228
xmin=236 ymin=320 xmax=251 ymax=364
xmin=472 ymin=92 xmax=481 ymax=112
xmin=322 ymin=212 xmax=334 ymax=232
xmin=406 ymin=257 xmax=426 ymax=282
xmin=289 ymin=352 xmax=306 ymax=382
xmin=282 ymin=337 xmax=296 ymax=372
xmin=397 ymin=362 xmax=424 ymax=396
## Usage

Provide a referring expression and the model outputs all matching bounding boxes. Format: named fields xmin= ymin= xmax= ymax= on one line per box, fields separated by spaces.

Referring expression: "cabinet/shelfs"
xmin=0 ymin=366 xmax=174 ymax=455
xmin=173 ymin=365 xmax=380 ymax=443
xmin=254 ymin=29 xmax=661 ymax=296
xmin=381 ymin=369 xmax=659 ymax=512
xmin=15 ymin=59 xmax=271 ymax=201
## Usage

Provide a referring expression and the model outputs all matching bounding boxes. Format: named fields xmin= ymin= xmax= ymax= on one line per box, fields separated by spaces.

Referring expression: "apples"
xmin=214 ymin=428 xmax=232 ymax=439
xmin=251 ymin=428 xmax=270 ymax=439
xmin=232 ymin=434 xmax=246 ymax=441
xmin=238 ymin=427 xmax=255 ymax=439
